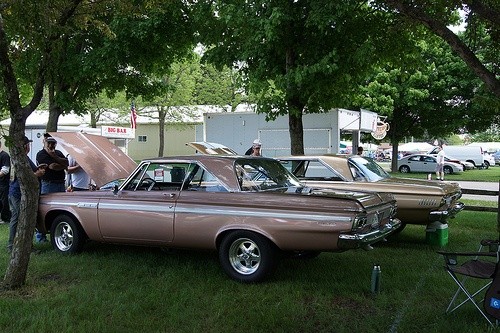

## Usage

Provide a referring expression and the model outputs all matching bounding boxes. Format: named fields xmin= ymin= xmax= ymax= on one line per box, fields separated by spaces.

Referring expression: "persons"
xmin=64 ymin=153 xmax=90 ymax=191
xmin=35 ymin=137 xmax=69 ymax=242
xmin=245 ymin=139 xmax=262 ymax=156
xmin=0 ymin=136 xmax=45 ymax=255
xmin=436 ymin=147 xmax=445 ymax=181
xmin=358 ymin=147 xmax=413 ymax=161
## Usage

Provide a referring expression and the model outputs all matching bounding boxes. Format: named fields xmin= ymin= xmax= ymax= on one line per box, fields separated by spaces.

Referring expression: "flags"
xmin=130 ymin=99 xmax=137 ymax=129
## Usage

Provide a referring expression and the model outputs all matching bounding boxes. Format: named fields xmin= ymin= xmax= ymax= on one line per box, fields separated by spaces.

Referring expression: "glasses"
xmin=253 ymin=148 xmax=260 ymax=149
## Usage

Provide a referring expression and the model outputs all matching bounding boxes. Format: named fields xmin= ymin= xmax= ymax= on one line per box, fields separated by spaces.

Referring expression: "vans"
xmin=428 ymin=145 xmax=484 ymax=167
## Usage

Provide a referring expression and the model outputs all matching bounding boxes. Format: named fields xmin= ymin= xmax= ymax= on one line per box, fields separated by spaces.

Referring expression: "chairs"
xmin=438 ymin=240 xmax=500 ymax=330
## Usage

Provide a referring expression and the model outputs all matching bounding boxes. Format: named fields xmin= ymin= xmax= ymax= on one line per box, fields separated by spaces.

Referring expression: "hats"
xmin=253 ymin=139 xmax=263 ymax=145
xmin=24 ymin=136 xmax=33 ymax=145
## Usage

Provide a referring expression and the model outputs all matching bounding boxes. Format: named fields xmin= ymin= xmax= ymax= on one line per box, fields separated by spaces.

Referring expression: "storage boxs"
xmin=426 ymin=222 xmax=448 ymax=247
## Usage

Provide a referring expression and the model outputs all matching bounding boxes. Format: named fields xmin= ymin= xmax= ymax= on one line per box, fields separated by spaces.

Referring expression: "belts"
xmin=42 ymin=180 xmax=66 ymax=184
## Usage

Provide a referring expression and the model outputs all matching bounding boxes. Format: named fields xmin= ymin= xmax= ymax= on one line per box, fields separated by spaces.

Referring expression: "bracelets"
xmin=33 ymin=172 xmax=37 ymax=175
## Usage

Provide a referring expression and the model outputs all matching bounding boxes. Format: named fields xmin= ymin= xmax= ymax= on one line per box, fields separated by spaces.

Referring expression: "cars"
xmin=36 ymin=131 xmax=401 ymax=282
xmin=395 ymin=154 xmax=465 ymax=174
xmin=443 ymin=155 xmax=475 ymax=170
xmin=185 ymin=136 xmax=465 ymax=225
xmin=480 ymin=153 xmax=496 ymax=169
xmin=493 ymin=150 xmax=500 ymax=166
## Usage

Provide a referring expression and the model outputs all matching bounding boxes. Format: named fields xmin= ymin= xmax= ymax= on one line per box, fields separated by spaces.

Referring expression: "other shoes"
xmin=7 ymin=246 xmax=12 ymax=254
xmin=30 ymin=248 xmax=40 ymax=254
xmin=0 ymin=218 xmax=10 ymax=224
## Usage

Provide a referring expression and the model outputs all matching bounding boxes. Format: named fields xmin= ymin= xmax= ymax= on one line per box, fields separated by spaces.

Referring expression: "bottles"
xmin=371 ymin=266 xmax=381 ymax=295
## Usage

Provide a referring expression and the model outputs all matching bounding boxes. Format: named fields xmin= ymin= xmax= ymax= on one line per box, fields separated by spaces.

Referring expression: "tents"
xmin=339 ymin=141 xmax=433 ymax=153
